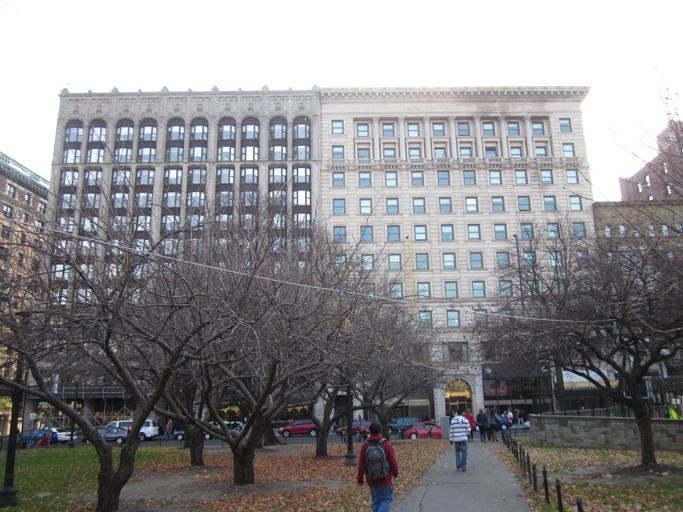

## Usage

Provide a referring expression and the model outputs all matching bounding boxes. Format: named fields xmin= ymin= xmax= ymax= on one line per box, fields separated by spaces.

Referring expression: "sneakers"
xmin=457 ymin=465 xmax=466 ymax=471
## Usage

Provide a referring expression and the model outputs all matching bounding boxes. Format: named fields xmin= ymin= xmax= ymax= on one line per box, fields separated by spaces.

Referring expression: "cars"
xmin=278 ymin=420 xmax=317 ymax=437
xmin=472 ymin=416 xmax=511 ymax=432
xmin=15 ymin=428 xmax=78 ymax=442
xmin=168 ymin=420 xmax=244 ymax=441
xmin=336 ymin=416 xmax=442 ymax=439
xmin=79 ymin=425 xmax=127 ymax=444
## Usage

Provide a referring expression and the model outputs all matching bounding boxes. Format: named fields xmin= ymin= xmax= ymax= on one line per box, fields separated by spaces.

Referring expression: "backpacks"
xmin=362 ymin=437 xmax=391 ymax=482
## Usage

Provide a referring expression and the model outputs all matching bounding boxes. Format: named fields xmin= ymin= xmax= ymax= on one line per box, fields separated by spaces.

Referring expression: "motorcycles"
xmin=341 ymin=429 xmax=367 ymax=443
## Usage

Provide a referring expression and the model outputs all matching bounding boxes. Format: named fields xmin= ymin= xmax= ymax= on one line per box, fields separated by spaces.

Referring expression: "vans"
xmin=105 ymin=419 xmax=159 ymax=440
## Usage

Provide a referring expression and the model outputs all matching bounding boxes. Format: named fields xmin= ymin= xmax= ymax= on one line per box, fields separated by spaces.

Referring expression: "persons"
xmin=331 ymin=414 xmax=368 ymax=444
xmin=445 ymin=406 xmax=525 ymax=443
xmin=447 ymin=407 xmax=472 ymax=473
xmin=356 ymin=421 xmax=400 ymax=512
xmin=36 ymin=433 xmax=48 ymax=446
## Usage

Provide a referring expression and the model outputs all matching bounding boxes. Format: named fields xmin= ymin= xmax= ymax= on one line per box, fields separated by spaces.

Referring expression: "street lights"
xmin=69 ymin=375 xmax=80 ymax=443
